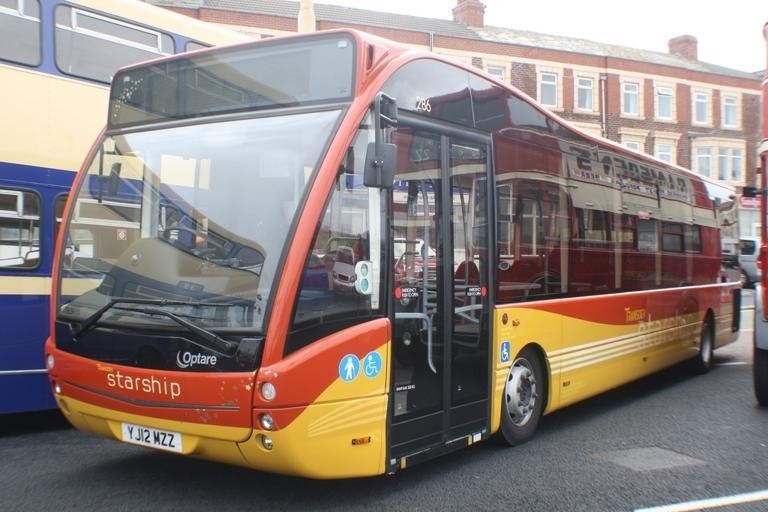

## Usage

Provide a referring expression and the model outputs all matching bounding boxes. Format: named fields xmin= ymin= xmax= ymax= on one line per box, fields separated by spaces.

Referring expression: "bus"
xmin=60 ymin=132 xmax=265 ymax=339
xmin=43 ymin=28 xmax=745 ymax=481
xmin=0 ymin=0 xmax=271 ymax=419
xmin=743 ymin=20 xmax=768 ymax=412
xmin=477 ymin=125 xmax=722 ymax=302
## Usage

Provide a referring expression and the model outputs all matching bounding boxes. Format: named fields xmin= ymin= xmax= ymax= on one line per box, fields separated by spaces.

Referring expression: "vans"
xmin=739 ymin=238 xmax=760 ymax=287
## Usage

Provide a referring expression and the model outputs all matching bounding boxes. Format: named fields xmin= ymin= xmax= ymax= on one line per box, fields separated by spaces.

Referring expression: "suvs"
xmin=331 ymin=244 xmax=355 ymax=297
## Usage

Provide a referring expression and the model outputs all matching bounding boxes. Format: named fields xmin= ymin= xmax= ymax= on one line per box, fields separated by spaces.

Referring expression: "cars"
xmin=392 ymin=237 xmax=437 ymax=277
